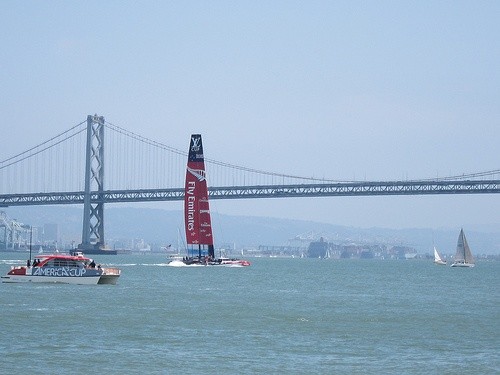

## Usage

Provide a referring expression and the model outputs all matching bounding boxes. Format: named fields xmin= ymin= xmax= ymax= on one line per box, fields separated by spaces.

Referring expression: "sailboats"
xmin=168 ymin=133 xmax=250 ymax=267
xmin=450 ymin=228 xmax=475 ymax=267
xmin=433 ymin=246 xmax=447 ymax=265
xmin=167 ymin=227 xmax=186 ymax=259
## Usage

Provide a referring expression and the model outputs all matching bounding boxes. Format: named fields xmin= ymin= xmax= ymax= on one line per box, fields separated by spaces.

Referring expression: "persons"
xmin=32 ymin=259 xmax=37 ymax=267
xmin=98 ymin=264 xmax=101 ymax=268
xmin=26 ymin=260 xmax=31 ymax=266
xmin=37 ymin=259 xmax=41 ymax=263
xmin=87 ymin=260 xmax=95 ymax=268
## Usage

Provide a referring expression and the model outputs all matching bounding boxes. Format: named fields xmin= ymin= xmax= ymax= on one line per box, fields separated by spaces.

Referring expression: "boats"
xmin=1 ymin=226 xmax=121 ymax=285
xmin=70 ymin=247 xmax=83 ymax=258
